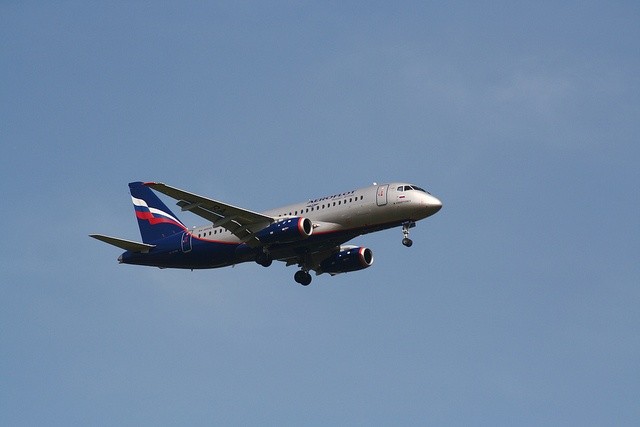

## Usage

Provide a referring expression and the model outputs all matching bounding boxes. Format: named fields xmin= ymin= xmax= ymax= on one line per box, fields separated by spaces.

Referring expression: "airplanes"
xmin=90 ymin=182 xmax=442 ymax=285
